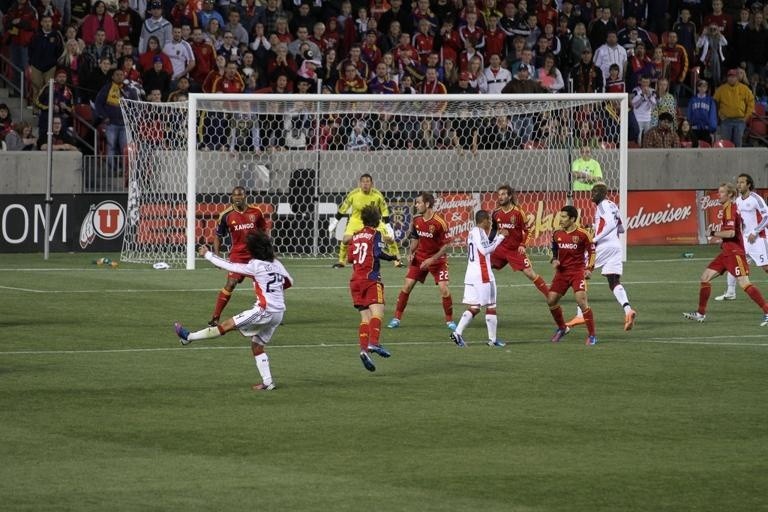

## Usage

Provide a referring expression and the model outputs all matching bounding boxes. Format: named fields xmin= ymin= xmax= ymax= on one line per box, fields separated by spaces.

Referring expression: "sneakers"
xmin=394 ymin=260 xmax=405 ymax=269
xmin=388 ymin=316 xmax=401 ymax=328
xmin=446 ymin=320 xmax=457 ymax=331
xmin=681 ymin=310 xmax=705 ymax=324
xmin=714 ymin=293 xmax=736 ymax=301
xmin=332 ymin=262 xmax=345 ymax=268
xmin=251 ymin=383 xmax=276 ymax=391
xmin=208 ymin=316 xmax=220 ymax=327
xmin=174 ymin=321 xmax=192 ymax=346
xmin=367 ymin=343 xmax=391 ymax=358
xmin=624 ymin=309 xmax=636 ymax=331
xmin=551 ymin=317 xmax=598 ymax=347
xmin=487 ymin=339 xmax=506 ymax=348
xmin=359 ymin=349 xmax=376 ymax=372
xmin=450 ymin=331 xmax=468 ymax=348
xmin=760 ymin=314 xmax=768 ymax=327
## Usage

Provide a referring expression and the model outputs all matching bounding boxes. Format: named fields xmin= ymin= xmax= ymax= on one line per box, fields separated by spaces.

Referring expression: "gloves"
xmin=384 ymin=223 xmax=395 ymax=240
xmin=328 ymin=217 xmax=339 ymax=233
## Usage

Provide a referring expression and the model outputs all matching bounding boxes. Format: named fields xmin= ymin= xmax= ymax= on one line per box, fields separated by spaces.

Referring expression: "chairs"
xmin=599 ymin=138 xmax=733 ymax=149
xmin=750 ymin=104 xmax=768 ymax=137
xmin=74 ymin=104 xmax=163 ymax=153
xmin=646 ymin=31 xmax=667 ymax=47
